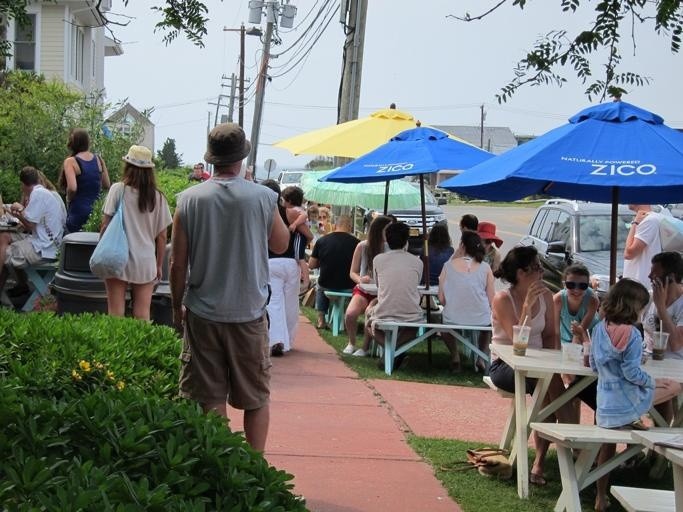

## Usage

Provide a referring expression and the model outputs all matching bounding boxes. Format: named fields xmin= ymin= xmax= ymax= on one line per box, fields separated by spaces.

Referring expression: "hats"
xmin=477 ymin=221 xmax=503 ymax=248
xmin=203 ymin=122 xmax=252 ymax=165
xmin=121 ymin=144 xmax=155 ymax=168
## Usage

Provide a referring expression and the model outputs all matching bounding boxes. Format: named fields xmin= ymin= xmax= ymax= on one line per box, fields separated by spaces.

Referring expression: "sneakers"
xmin=343 ymin=343 xmax=355 ymax=354
xmin=352 ymin=348 xmax=369 ymax=357
xmin=271 ymin=342 xmax=284 ymax=357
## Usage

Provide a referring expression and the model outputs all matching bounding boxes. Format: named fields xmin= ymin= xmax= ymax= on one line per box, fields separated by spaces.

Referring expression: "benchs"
xmin=375 ymin=321 xmax=493 ymax=375
xmin=529 ymin=422 xmax=683 ymax=512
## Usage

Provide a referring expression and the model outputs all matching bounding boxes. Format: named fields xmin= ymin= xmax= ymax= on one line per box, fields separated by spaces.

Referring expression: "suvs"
xmin=374 ymin=181 xmax=447 ymax=241
xmin=278 ymin=168 xmax=303 ymax=192
xmin=519 ymin=198 xmax=666 ymax=313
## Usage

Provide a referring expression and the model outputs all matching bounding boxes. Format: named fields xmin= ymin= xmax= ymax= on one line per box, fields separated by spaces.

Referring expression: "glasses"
xmin=564 ymin=281 xmax=589 ymax=291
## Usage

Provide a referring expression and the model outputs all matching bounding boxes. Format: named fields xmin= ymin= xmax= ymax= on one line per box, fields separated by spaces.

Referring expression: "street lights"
xmin=244 ymin=27 xmax=274 ymax=168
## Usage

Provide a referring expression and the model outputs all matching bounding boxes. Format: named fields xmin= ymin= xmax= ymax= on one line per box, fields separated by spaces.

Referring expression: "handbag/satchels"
xmin=440 ymin=446 xmax=512 ymax=481
xmin=89 ymin=191 xmax=129 ymax=278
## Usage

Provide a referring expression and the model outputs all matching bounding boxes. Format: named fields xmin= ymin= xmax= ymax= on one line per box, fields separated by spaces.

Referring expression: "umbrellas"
xmin=318 ymin=120 xmax=496 ymax=214
xmin=436 ymin=92 xmax=683 ymax=290
xmin=273 ymin=102 xmax=465 ymax=217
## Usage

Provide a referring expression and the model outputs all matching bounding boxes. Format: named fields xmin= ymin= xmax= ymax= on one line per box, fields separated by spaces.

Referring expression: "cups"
xmin=652 ymin=332 xmax=670 ymax=361
xmin=512 ymin=325 xmax=531 ymax=357
xmin=583 ymin=342 xmax=593 ymax=367
xmin=562 ymin=343 xmax=582 ymax=364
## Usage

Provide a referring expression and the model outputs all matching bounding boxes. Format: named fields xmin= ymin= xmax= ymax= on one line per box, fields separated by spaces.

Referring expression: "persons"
xmin=188 ymin=162 xmax=210 ymax=181
xmin=622 ymin=205 xmax=661 ymax=290
xmin=98 ymin=144 xmax=173 ymax=321
xmin=639 ymin=251 xmax=683 ymax=354
xmin=365 ymin=222 xmax=423 ymax=369
xmin=343 ymin=215 xmax=392 ymax=356
xmin=489 ymin=246 xmax=582 ymax=485
xmin=309 ymin=215 xmax=361 ymax=329
xmin=477 ymin=222 xmax=503 ymax=272
xmin=258 ymin=179 xmax=314 ymax=356
xmin=0 ymin=165 xmax=67 ymax=289
xmin=305 ymin=206 xmax=332 ymax=248
xmin=438 ymin=231 xmax=495 ymax=373
xmin=459 ymin=214 xmax=478 ymax=233
xmin=552 ymin=264 xmax=599 ymax=344
xmin=589 ymin=277 xmax=681 ymax=430
xmin=64 ymin=129 xmax=111 ymax=232
xmin=427 ymin=223 xmax=454 ymax=285
xmin=169 ymin=123 xmax=289 ymax=452
xmin=280 ymin=185 xmax=310 ymax=288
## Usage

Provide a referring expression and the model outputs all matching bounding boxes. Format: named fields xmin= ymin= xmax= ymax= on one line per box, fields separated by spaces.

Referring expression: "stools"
xmin=22 ymin=261 xmax=60 ymax=313
xmin=322 ymin=289 xmax=353 ymax=337
xmin=609 ymin=484 xmax=676 ymax=512
xmin=482 ymin=375 xmax=516 ymax=452
